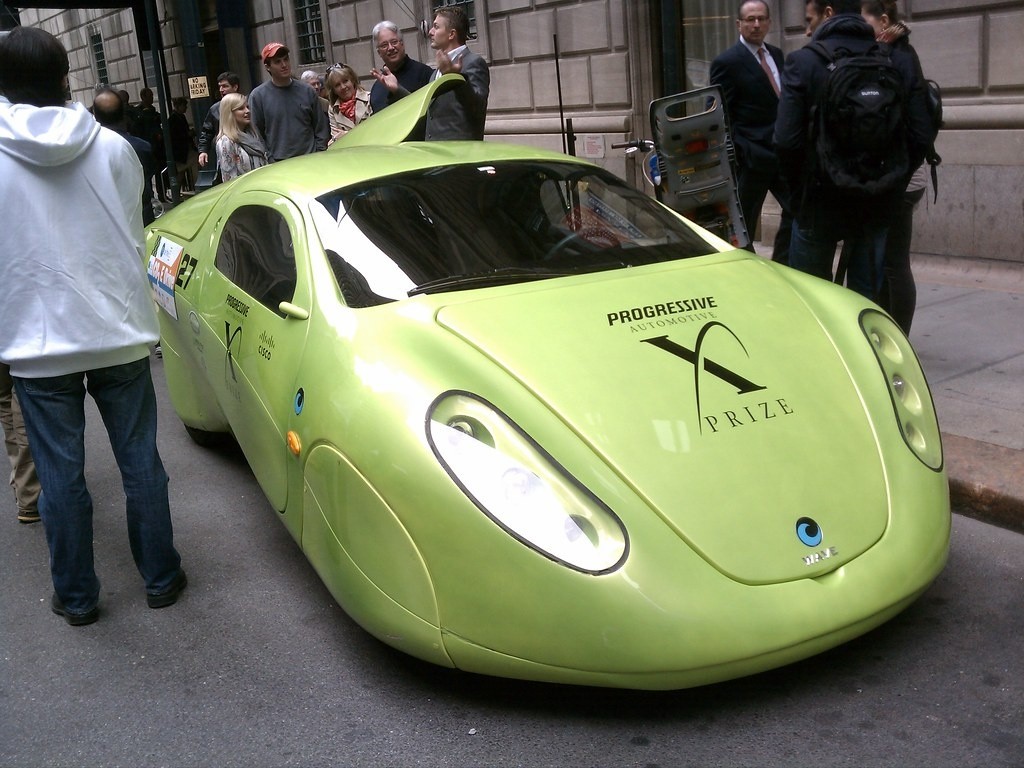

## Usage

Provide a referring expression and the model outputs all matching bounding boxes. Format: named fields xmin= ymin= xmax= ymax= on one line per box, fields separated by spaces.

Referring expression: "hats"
xmin=261 ymin=43 xmax=290 ymax=63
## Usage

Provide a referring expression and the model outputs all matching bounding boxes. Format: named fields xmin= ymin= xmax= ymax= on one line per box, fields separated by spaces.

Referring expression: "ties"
xmin=757 ymin=49 xmax=780 ymax=100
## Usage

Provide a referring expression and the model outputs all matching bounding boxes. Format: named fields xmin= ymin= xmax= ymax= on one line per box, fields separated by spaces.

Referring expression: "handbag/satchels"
xmin=923 ymin=78 xmax=942 ymax=167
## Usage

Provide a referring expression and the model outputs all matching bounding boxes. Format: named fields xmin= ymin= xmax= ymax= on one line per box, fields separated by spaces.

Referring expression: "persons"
xmin=0 ymin=362 xmax=41 ymax=522
xmin=301 ymin=70 xmax=330 ymax=115
xmin=91 ymin=88 xmax=199 ymax=228
xmin=370 ymin=21 xmax=436 ymax=141
xmin=706 ymin=0 xmax=794 ymax=266
xmin=324 ymin=64 xmax=373 ymax=146
xmin=370 ymin=6 xmax=490 ymax=141
xmin=0 ymin=25 xmax=188 ymax=626
xmin=861 ymin=0 xmax=924 ymax=337
xmin=772 ymin=0 xmax=933 ymax=299
xmin=248 ymin=43 xmax=328 ymax=165
xmin=197 ymin=72 xmax=241 ymax=187
xmin=216 ymin=93 xmax=269 ymax=183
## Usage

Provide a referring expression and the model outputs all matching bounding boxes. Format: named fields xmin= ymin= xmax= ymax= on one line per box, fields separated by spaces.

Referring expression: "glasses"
xmin=378 ymin=40 xmax=400 ymax=48
xmin=738 ymin=17 xmax=769 ymax=24
xmin=326 ymin=62 xmax=346 ymax=73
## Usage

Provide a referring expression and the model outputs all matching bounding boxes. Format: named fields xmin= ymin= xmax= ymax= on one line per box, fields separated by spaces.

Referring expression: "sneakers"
xmin=147 ymin=566 xmax=187 ymax=609
xmin=51 ymin=591 xmax=101 ymax=627
xmin=17 ymin=507 xmax=46 ymax=522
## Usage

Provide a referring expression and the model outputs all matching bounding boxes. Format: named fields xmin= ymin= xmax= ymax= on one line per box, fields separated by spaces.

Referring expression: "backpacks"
xmin=801 ymin=38 xmax=904 ymax=202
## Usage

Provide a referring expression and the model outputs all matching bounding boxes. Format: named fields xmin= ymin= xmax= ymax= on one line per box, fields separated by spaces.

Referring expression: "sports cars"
xmin=141 ymin=71 xmax=950 ymax=690
